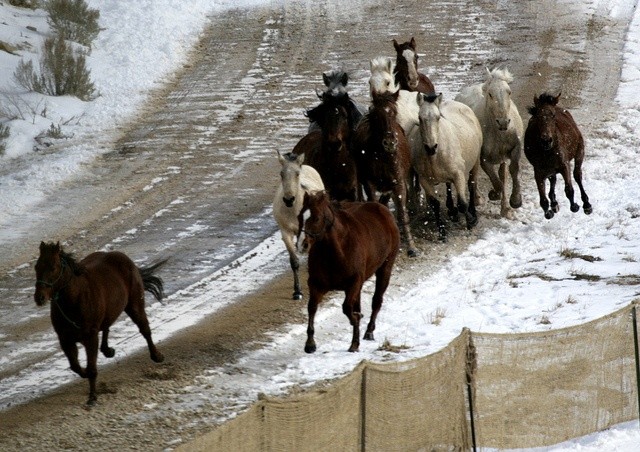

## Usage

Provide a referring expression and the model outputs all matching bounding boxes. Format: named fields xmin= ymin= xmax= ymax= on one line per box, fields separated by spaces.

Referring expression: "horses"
xmin=295 ymin=188 xmax=401 ymax=353
xmin=368 ymin=56 xmax=429 ymax=214
xmin=273 ymin=149 xmax=326 ymax=300
xmin=454 ymin=64 xmax=524 ymax=219
xmin=407 ymin=90 xmax=483 ymax=243
xmin=292 ymin=88 xmax=358 ymax=202
xmin=322 ymin=68 xmax=373 ymax=133
xmin=34 ymin=241 xmax=173 ymax=405
xmin=392 ymin=37 xmax=435 ymax=96
xmin=523 ymin=88 xmax=592 ymax=219
xmin=354 ymin=87 xmax=420 ymax=258
